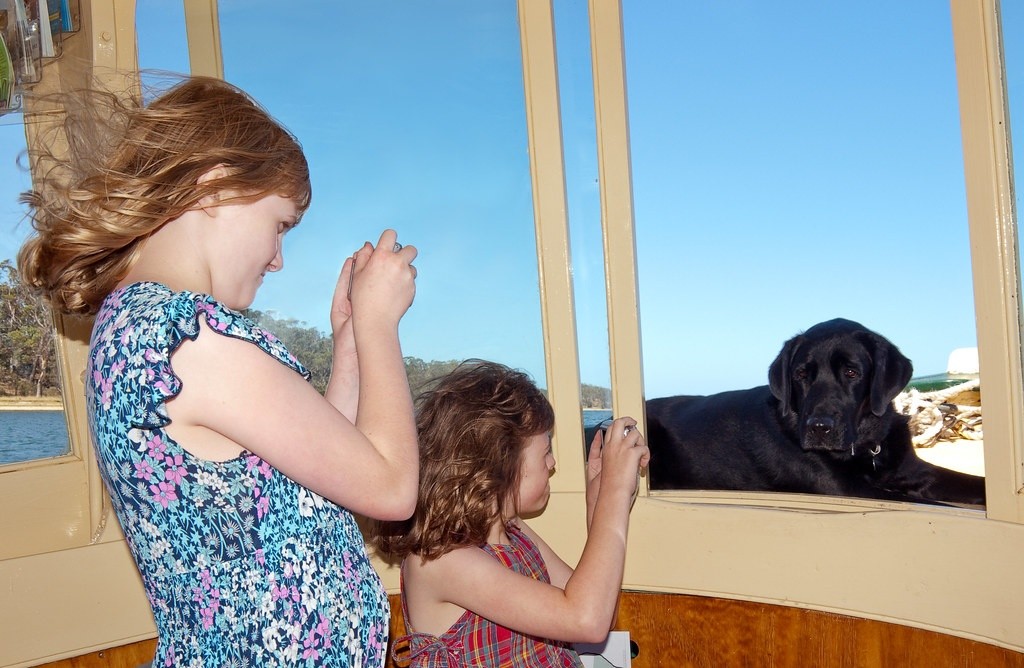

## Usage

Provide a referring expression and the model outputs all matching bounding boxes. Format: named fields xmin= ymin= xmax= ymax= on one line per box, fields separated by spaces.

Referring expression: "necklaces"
xmin=16 ymin=75 xmax=422 ymax=668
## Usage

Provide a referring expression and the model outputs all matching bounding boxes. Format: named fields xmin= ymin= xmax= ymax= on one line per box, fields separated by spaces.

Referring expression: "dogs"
xmin=646 ymin=316 xmax=985 ymax=507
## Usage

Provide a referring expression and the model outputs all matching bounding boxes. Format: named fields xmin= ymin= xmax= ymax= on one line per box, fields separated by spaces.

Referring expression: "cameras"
xmin=601 ymin=421 xmax=631 ymax=445
xmin=348 ymin=243 xmax=402 ymax=301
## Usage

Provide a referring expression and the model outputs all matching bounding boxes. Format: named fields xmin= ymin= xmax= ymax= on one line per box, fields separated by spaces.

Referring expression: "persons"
xmin=369 ymin=357 xmax=650 ymax=668
